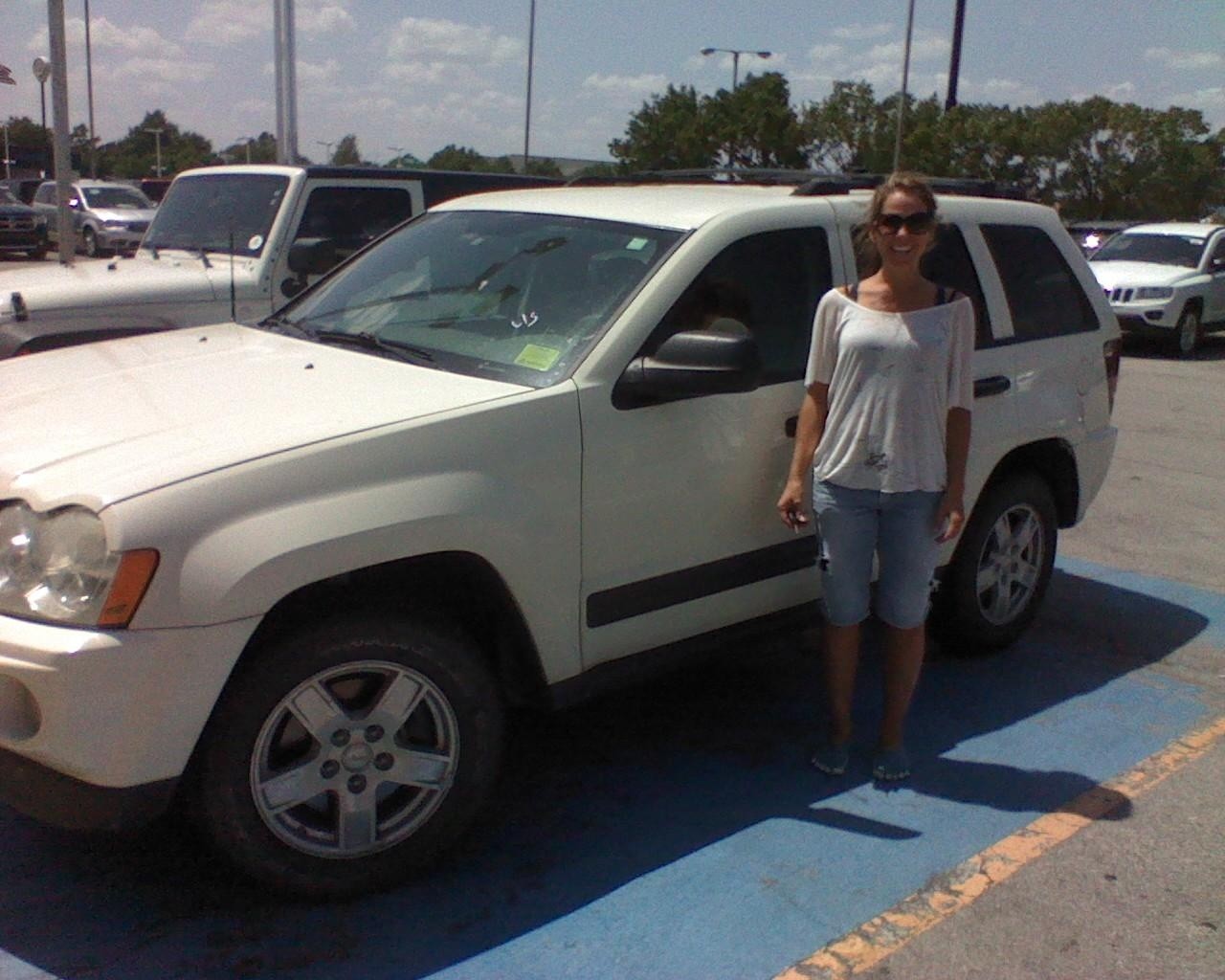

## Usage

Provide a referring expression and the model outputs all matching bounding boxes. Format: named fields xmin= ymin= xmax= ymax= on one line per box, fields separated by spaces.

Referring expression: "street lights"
xmin=144 ymin=127 xmax=167 ymax=177
xmin=702 ymin=47 xmax=774 ymax=183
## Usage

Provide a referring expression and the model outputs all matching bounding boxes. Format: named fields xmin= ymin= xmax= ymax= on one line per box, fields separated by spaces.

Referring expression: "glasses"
xmin=870 ymin=212 xmax=933 ymax=237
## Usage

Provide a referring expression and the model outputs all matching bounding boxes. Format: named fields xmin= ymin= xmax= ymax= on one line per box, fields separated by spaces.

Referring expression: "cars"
xmin=1069 ymin=218 xmax=1225 ymax=357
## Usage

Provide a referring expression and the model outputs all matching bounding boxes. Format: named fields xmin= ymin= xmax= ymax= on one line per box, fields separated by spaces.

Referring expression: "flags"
xmin=0 ymin=65 xmax=17 ymax=85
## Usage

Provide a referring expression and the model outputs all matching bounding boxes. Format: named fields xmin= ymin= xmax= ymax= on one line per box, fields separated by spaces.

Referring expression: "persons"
xmin=675 ymin=276 xmax=753 ymax=339
xmin=779 ymin=178 xmax=975 ymax=779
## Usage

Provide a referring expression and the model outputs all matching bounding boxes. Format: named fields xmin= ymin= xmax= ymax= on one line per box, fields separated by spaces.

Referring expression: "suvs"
xmin=0 ymin=176 xmax=178 ymax=258
xmin=1 ymin=170 xmax=1127 ymax=904
xmin=0 ymin=165 xmax=573 ymax=357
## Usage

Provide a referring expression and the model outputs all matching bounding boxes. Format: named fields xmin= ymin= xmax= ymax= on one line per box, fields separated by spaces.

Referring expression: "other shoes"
xmin=873 ymin=747 xmax=911 ymax=781
xmin=809 ymin=735 xmax=850 ymax=775
